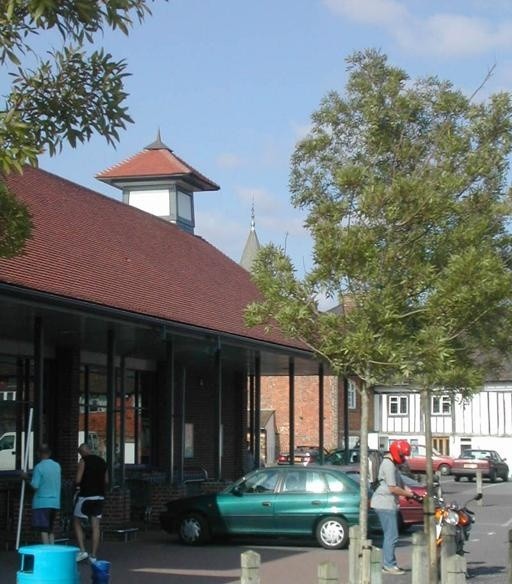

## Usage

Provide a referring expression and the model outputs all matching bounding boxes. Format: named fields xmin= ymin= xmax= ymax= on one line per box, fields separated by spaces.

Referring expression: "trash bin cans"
xmin=16 ymin=544 xmax=81 ymax=584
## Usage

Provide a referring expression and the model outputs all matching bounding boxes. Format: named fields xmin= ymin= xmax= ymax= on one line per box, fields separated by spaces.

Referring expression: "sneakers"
xmin=76 ymin=551 xmax=97 ymax=566
xmin=381 ymin=565 xmax=404 ymax=576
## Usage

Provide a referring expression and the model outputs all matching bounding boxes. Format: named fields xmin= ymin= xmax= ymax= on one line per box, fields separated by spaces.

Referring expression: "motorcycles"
xmin=421 ymin=492 xmax=483 ymax=580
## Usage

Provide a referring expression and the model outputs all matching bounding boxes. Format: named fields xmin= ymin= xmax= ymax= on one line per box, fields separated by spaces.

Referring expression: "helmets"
xmin=390 ymin=440 xmax=412 ymax=464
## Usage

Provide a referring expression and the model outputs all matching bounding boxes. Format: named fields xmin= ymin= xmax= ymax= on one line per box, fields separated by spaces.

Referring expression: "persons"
xmin=369 ymin=440 xmax=417 ymax=574
xmin=21 ymin=442 xmax=62 ymax=544
xmin=72 ymin=441 xmax=107 ymax=564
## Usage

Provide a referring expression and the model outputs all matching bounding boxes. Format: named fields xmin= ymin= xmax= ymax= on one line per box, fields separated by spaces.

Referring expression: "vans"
xmin=0 ymin=431 xmax=101 ymax=472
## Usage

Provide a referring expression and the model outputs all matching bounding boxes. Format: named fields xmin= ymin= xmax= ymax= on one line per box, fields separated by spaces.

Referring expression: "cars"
xmin=276 ymin=444 xmax=509 ymax=483
xmin=158 ymin=463 xmax=440 ymax=551
